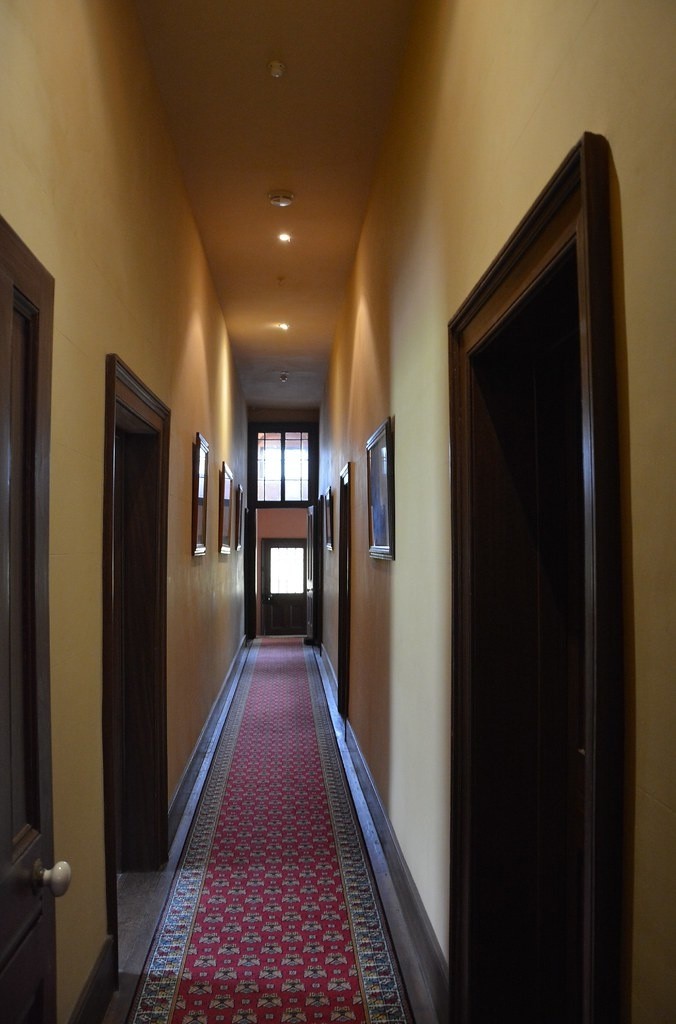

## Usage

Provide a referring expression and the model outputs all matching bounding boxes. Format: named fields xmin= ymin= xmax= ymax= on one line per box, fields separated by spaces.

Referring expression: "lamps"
xmin=269 ymin=61 xmax=286 ymax=79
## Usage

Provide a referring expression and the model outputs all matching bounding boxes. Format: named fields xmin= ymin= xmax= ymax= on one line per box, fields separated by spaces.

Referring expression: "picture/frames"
xmin=235 ymin=483 xmax=244 ymax=552
xmin=366 ymin=416 xmax=395 ymax=560
xmin=192 ymin=432 xmax=210 ymax=557
xmin=325 ymin=487 xmax=334 ymax=551
xmin=219 ymin=461 xmax=234 ymax=555
